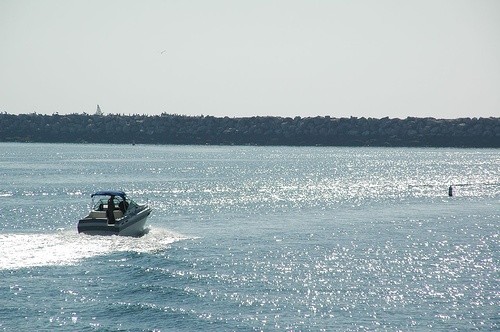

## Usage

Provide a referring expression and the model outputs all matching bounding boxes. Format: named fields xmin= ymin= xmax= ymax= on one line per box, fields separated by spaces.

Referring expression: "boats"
xmin=77 ymin=190 xmax=153 ymax=237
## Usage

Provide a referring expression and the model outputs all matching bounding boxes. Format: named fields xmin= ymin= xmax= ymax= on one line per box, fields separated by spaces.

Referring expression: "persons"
xmin=119 ymin=197 xmax=129 ymax=214
xmin=108 ymin=196 xmax=115 ymax=224
xmin=97 ymin=204 xmax=103 ymax=211
